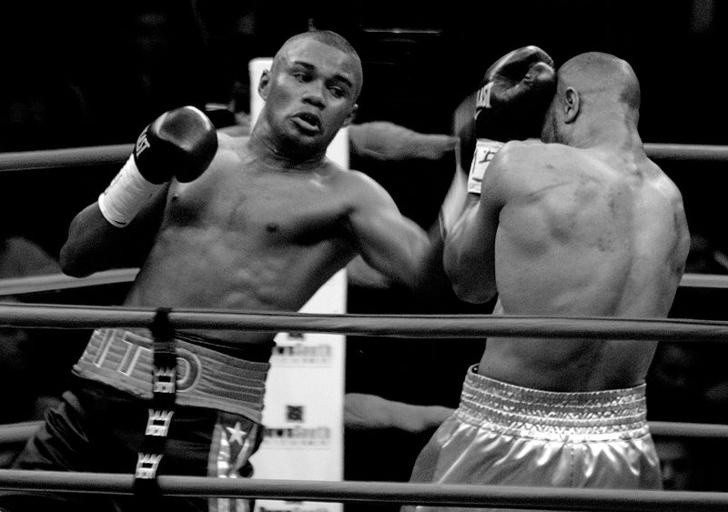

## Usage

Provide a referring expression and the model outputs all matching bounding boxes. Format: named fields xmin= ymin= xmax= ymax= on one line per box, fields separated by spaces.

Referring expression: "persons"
xmin=653 ymin=433 xmax=694 ymax=491
xmin=0 ymin=27 xmax=483 ymax=511
xmin=0 ymin=235 xmax=63 ymax=356
xmin=399 ymin=44 xmax=693 ymax=509
